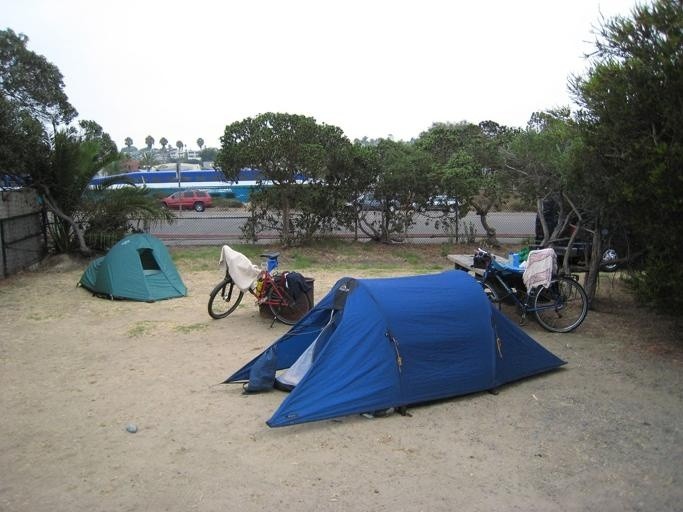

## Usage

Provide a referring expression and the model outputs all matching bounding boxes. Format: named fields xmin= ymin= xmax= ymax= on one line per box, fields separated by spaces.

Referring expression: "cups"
xmin=256 ymin=278 xmax=264 ymax=296
xmin=513 ymin=254 xmax=520 ymax=268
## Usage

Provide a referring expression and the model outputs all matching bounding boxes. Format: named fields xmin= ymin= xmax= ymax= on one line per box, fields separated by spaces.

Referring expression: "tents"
xmin=219 ymin=269 xmax=567 ymax=427
xmin=79 ymin=234 xmax=187 ymax=302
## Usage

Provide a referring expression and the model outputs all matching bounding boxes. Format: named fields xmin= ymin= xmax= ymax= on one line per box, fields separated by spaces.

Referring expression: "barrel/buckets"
xmin=257 ymin=272 xmax=280 ymax=318
xmin=280 ymin=275 xmax=315 ymax=322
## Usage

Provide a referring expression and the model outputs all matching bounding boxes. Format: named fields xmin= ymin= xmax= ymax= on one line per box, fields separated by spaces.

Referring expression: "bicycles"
xmin=208 ymin=244 xmax=314 ymax=328
xmin=473 ymin=247 xmax=588 ymax=333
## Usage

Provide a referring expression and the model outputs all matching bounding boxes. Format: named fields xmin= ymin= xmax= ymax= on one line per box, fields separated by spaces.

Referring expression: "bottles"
xmin=474 ymin=250 xmax=491 ymax=269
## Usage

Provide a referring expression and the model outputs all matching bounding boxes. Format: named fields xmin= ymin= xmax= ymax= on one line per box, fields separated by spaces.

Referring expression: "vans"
xmin=534 ymin=193 xmax=621 ymax=273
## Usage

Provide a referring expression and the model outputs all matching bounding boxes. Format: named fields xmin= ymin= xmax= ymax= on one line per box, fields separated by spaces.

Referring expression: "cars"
xmin=344 ymin=193 xmax=460 ymax=213
xmin=162 ymin=189 xmax=211 ymax=212
